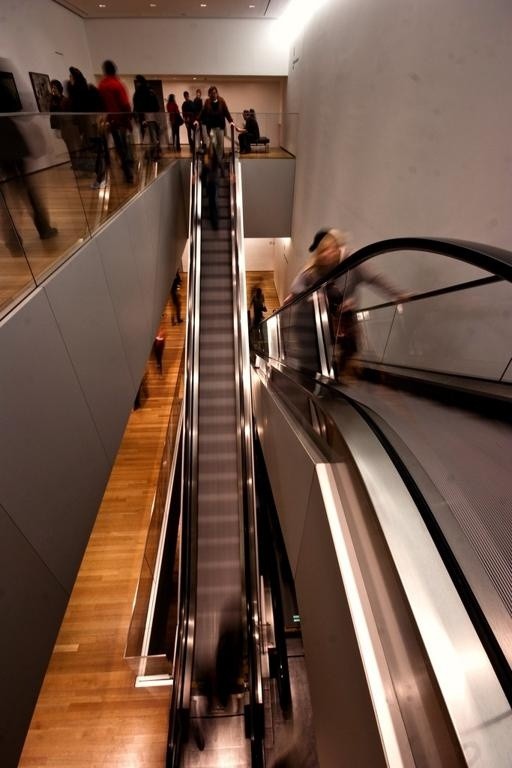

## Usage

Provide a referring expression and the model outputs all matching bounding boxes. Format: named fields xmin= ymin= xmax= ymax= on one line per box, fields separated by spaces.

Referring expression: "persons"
xmin=151 ymin=330 xmax=170 ymax=377
xmin=236 ymin=108 xmax=259 ymax=154
xmin=248 ymin=277 xmax=266 ymax=327
xmin=132 ymin=75 xmax=162 ymax=160
xmin=198 ymin=152 xmax=219 ymax=230
xmin=283 ymin=226 xmax=408 ymax=386
xmin=131 ymin=372 xmax=151 ymax=411
xmin=166 ymin=94 xmax=180 ymax=149
xmin=182 ymin=86 xmax=235 ymax=160
xmin=169 ymin=272 xmax=184 ymax=326
xmin=48 ymin=60 xmax=135 ymax=188
xmin=2 ymin=85 xmax=58 ymax=254
